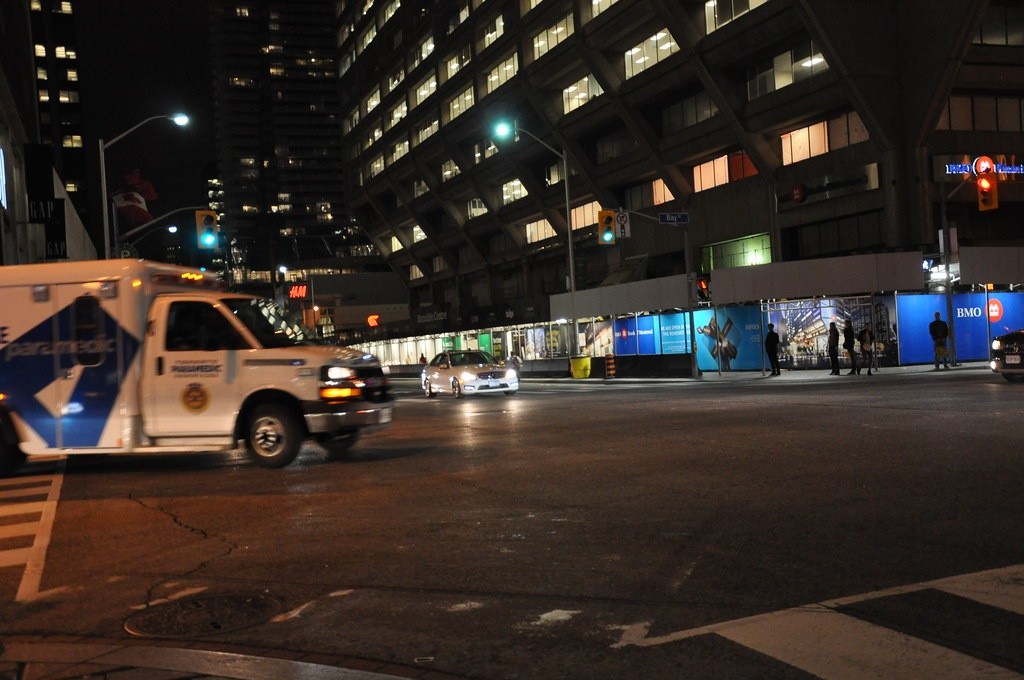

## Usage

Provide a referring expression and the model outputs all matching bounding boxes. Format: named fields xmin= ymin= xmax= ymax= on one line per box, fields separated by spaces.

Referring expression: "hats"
xmin=768 ymin=323 xmax=774 ymax=327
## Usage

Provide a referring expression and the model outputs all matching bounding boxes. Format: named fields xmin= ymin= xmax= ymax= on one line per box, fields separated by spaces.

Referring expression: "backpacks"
xmin=511 ymin=356 xmax=522 ymax=368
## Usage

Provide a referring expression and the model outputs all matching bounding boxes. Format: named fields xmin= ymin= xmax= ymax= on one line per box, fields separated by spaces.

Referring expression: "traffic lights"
xmin=599 ymin=210 xmax=617 ymax=245
xmin=195 ymin=211 xmax=219 ymax=250
xmin=695 ymin=276 xmax=709 ymax=306
xmin=978 ymin=170 xmax=998 ymax=210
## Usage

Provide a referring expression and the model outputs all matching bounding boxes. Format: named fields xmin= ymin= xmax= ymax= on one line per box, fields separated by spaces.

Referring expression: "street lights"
xmin=494 ymin=123 xmax=581 ymax=358
xmin=131 ymin=223 xmax=177 ymax=246
xmin=279 ymin=266 xmax=317 ymax=338
xmin=99 ymin=113 xmax=189 ymax=259
xmin=936 ymin=277 xmax=991 ymax=363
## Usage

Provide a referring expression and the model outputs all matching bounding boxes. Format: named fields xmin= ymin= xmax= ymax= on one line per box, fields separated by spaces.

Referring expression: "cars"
xmin=990 ymin=328 xmax=1024 ymax=383
xmin=420 ymin=348 xmax=520 ymax=398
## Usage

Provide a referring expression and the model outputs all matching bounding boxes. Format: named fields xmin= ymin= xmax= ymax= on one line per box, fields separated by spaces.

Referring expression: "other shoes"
xmin=857 ymin=370 xmax=860 ymax=376
xmin=944 ymin=365 xmax=949 ymax=368
xmin=777 ymin=372 xmax=780 ymax=375
xmin=936 ymin=365 xmax=939 ymax=370
xmin=829 ymin=371 xmax=839 ymax=375
xmin=847 ymin=371 xmax=855 ymax=375
xmin=771 ymin=372 xmax=776 ymax=375
xmin=868 ymin=371 xmax=872 ymax=375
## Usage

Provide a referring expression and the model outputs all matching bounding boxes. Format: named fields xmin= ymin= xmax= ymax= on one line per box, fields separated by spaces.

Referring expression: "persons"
xmin=765 ymin=323 xmax=780 ymax=375
xmin=509 ymin=351 xmax=523 ymax=385
xmin=828 ymin=320 xmax=875 ymax=377
xmin=419 ymin=353 xmax=426 ymax=364
xmin=929 ymin=312 xmax=949 ymax=370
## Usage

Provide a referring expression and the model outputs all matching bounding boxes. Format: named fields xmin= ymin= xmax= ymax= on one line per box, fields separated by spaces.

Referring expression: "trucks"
xmin=0 ymin=259 xmax=397 ymax=476
